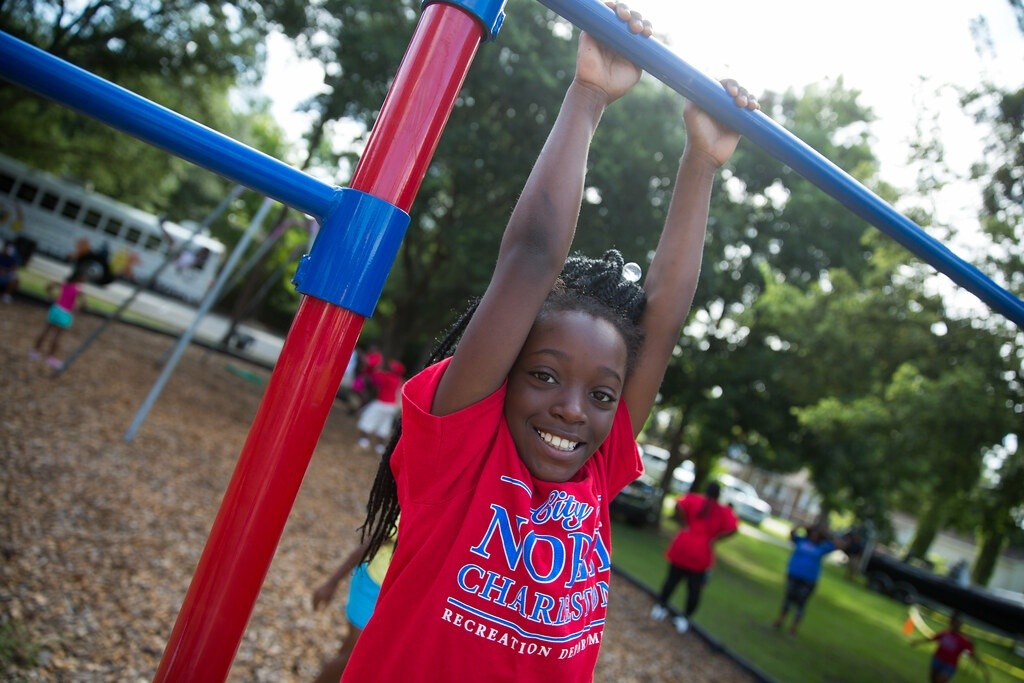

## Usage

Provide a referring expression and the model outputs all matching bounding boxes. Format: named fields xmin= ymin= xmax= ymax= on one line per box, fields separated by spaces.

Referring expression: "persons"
xmin=28 ymin=1 xmax=993 ymax=683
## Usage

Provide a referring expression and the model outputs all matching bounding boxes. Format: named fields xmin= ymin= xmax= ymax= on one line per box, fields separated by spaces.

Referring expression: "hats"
xmin=388 ymin=359 xmax=405 ymax=375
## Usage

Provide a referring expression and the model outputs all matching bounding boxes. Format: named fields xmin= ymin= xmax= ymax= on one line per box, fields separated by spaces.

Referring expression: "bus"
xmin=0 ymin=152 xmax=228 ymax=304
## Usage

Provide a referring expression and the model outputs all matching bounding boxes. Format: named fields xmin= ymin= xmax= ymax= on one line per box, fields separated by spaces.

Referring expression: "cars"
xmin=639 ymin=442 xmax=697 ymax=493
xmin=609 ymin=474 xmax=660 ymax=528
xmin=715 ymin=472 xmax=772 ymax=526
